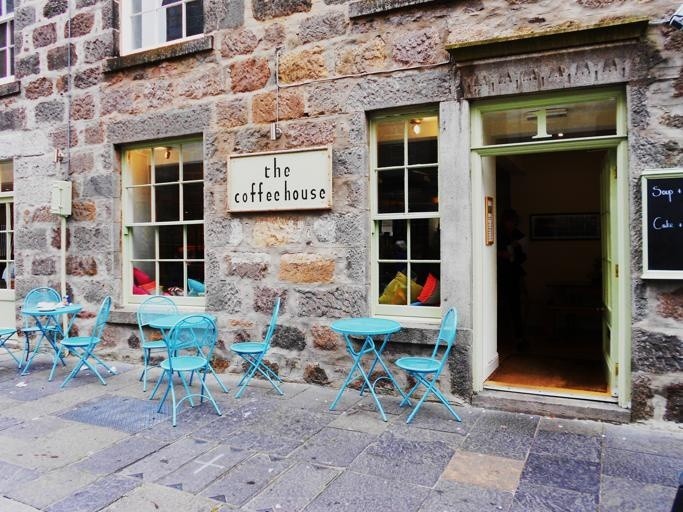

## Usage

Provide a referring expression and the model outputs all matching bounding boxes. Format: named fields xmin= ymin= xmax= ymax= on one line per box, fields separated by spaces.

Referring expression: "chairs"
xmin=0 ymin=329 xmax=24 ymax=368
xmin=60 ymin=296 xmax=117 ymax=388
xmin=137 ymin=296 xmax=179 ymax=392
xmin=158 ymin=314 xmax=216 ymax=427
xmin=229 ymin=295 xmax=284 ymax=398
xmin=394 ymin=306 xmax=462 ymax=424
xmin=18 ymin=287 xmax=62 ymax=369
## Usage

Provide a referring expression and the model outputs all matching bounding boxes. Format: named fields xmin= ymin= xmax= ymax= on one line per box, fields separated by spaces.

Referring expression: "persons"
xmin=396 ymin=237 xmax=407 ymax=250
xmin=497 ymin=206 xmax=526 ymax=341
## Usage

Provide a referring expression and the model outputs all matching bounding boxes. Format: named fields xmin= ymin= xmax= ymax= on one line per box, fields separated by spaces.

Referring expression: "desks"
xmin=149 ymin=313 xmax=228 ymax=413
xmin=21 ymin=304 xmax=83 ymax=381
xmin=330 ymin=318 xmax=402 ymax=422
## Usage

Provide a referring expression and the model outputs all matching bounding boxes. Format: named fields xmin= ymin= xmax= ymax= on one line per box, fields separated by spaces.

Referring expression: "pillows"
xmin=379 ymin=271 xmax=424 ymax=305
xmin=417 ymin=274 xmax=439 ymax=305
xmin=133 ymin=267 xmax=204 ymax=296
